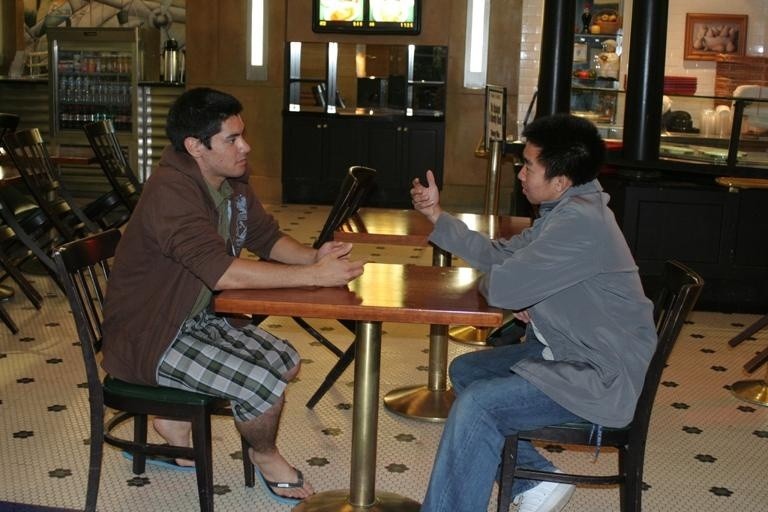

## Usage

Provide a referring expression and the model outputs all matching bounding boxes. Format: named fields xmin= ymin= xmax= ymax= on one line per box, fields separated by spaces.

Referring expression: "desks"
xmin=334 ymin=201 xmax=531 ymax=423
xmin=0 ymin=140 xmax=115 ymax=275
xmin=212 ymin=258 xmax=504 ymax=512
xmin=0 ymin=165 xmax=47 ymax=301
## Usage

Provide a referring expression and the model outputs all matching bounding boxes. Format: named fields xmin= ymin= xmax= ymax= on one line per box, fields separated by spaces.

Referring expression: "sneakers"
xmin=513 ymin=469 xmax=577 ymax=512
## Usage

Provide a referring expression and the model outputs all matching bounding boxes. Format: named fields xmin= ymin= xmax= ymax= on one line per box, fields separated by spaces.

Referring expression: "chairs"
xmin=0 ymin=127 xmax=103 ymax=333
xmin=255 ymin=163 xmax=392 ymax=407
xmin=0 ymin=113 xmax=20 ymax=149
xmin=728 ymin=315 xmax=768 ymax=373
xmin=52 ymin=227 xmax=256 ymax=512
xmin=81 ymin=119 xmax=143 ymax=232
xmin=499 ymin=258 xmax=704 ymax=511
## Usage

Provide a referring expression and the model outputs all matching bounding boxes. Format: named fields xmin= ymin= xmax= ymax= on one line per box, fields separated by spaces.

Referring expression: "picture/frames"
xmin=684 ymin=12 xmax=748 ymax=61
xmin=484 ymin=85 xmax=507 ymax=156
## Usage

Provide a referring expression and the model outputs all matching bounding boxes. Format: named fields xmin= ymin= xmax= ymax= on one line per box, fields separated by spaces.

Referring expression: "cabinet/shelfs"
xmin=626 ymin=189 xmax=767 ymax=314
xmin=571 ymin=32 xmax=620 ymax=94
xmin=283 ymin=107 xmax=446 ymax=210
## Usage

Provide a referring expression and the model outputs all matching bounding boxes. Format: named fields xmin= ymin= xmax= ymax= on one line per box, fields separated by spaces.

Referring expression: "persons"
xmin=101 ymin=86 xmax=364 ymax=505
xmin=580 ymin=2 xmax=592 ymax=34
xmin=596 ymin=38 xmax=620 ymax=80
xmin=410 ymin=114 xmax=657 ymax=511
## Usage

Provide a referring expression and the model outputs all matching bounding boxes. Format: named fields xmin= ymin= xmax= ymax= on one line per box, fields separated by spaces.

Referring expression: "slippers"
xmin=255 ymin=464 xmax=316 ymax=505
xmin=123 ymin=442 xmax=196 ymax=472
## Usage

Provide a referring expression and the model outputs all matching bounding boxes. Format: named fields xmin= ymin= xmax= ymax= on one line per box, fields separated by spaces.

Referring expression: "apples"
xmin=590 ymin=25 xmax=600 ymax=35
xmin=596 ymin=14 xmax=617 ymax=21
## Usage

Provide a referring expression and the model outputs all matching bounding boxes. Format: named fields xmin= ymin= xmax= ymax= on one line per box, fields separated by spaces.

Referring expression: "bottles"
xmin=59 ymin=55 xmax=131 ymax=132
xmin=579 ymin=8 xmax=592 ymax=35
xmin=162 ymin=39 xmax=179 ymax=83
